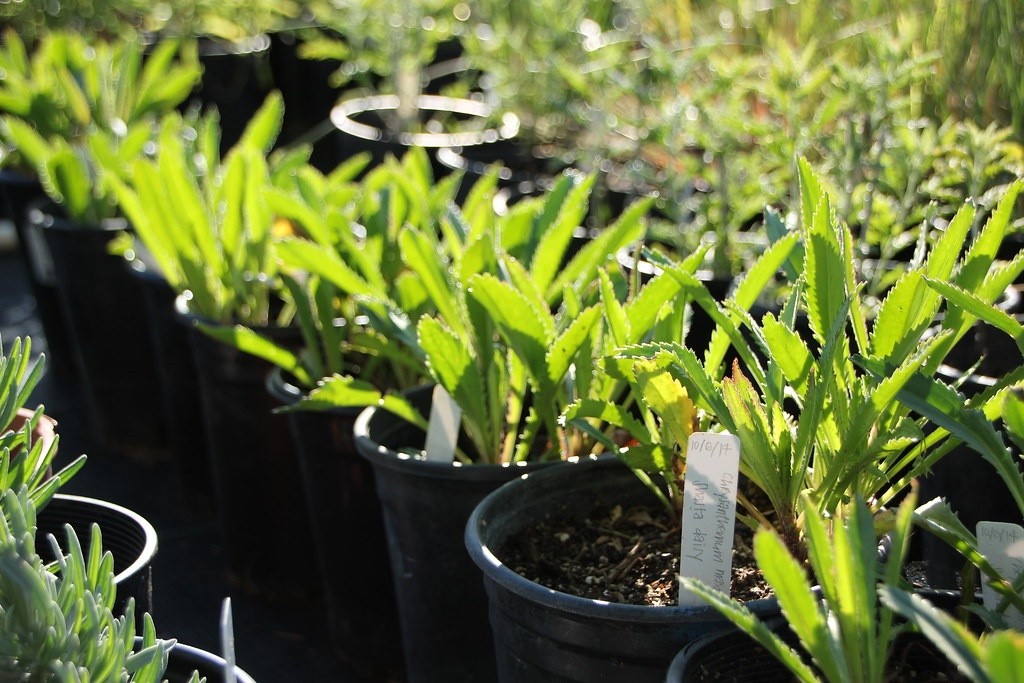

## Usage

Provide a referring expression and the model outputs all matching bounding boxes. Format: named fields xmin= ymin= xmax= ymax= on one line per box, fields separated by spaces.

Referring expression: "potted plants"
xmin=0 ymin=0 xmax=1023 ymax=681
